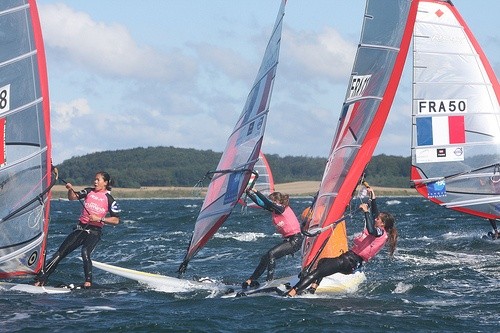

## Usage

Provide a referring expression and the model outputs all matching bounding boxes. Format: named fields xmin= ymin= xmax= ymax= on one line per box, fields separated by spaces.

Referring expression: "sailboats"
xmin=0 ymin=0 xmax=70 ymax=294
xmin=410 ymin=0 xmax=500 ymax=241
xmin=238 ymin=150 xmax=274 ymax=208
xmin=221 ymin=0 xmax=418 ymax=299
xmin=77 ymin=0 xmax=299 ymax=293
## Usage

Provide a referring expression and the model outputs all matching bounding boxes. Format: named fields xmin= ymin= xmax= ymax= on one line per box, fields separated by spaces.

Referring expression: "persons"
xmin=28 ymin=171 xmax=121 ymax=290
xmin=285 ymin=187 xmax=398 ymax=298
xmin=480 ymin=165 xmax=500 ymax=241
xmin=241 ymin=185 xmax=303 ymax=287
